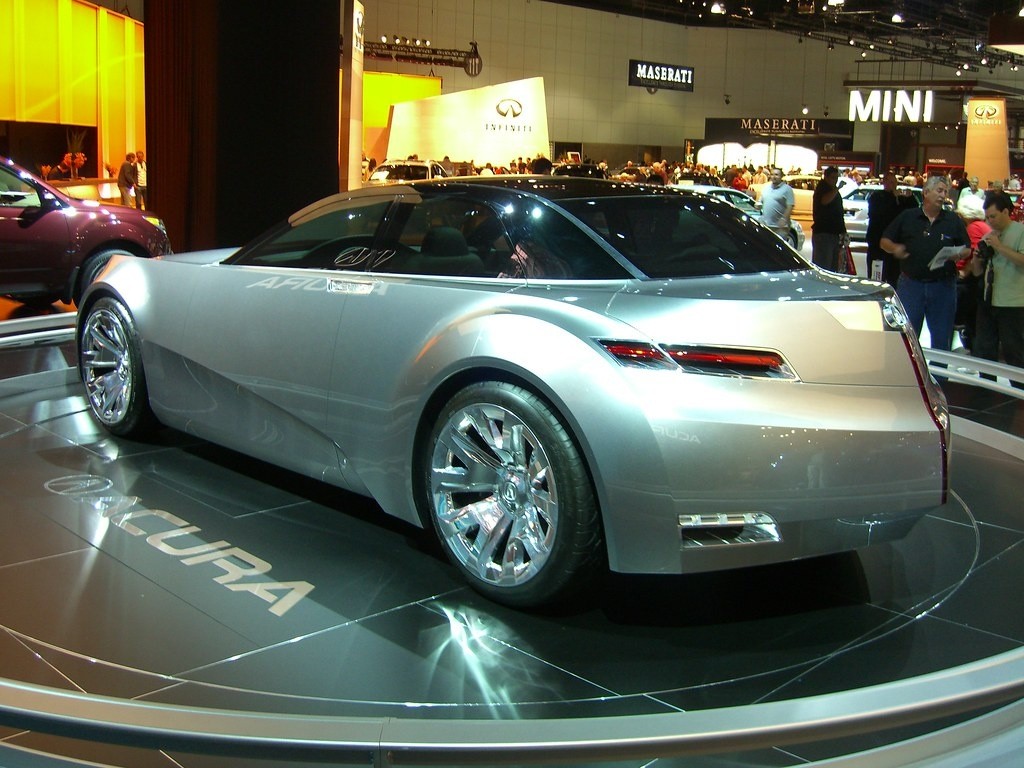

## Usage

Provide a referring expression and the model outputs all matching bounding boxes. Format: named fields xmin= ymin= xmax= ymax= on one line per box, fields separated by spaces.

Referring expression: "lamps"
xmin=981 ymin=55 xmax=987 ymax=64
xmin=892 ymin=10 xmax=905 ymax=23
xmin=412 ymin=38 xmax=420 ymax=45
xmin=711 ymin=0 xmax=726 ymax=15
xmin=402 ymin=36 xmax=410 ymax=44
xmin=1009 ymin=64 xmax=1019 ymax=71
xmin=956 ymin=63 xmax=962 ymax=76
xmin=828 ymin=0 xmax=845 ymax=11
xmin=963 ymin=59 xmax=970 ymax=70
xmin=422 ymin=39 xmax=431 ymax=46
xmin=381 ymin=35 xmax=388 ymax=44
xmin=393 ymin=35 xmax=400 ymax=44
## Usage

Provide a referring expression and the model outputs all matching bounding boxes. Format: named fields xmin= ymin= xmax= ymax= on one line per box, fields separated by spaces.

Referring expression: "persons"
xmin=844 ymin=169 xmax=879 ymax=185
xmin=408 ymin=154 xmax=418 ymax=161
xmin=811 ymin=166 xmax=848 ymax=272
xmin=967 ymin=189 xmax=1024 ymax=431
xmin=788 ymin=166 xmax=802 ymax=188
xmin=646 ymin=175 xmax=680 ymax=251
xmin=881 ymin=176 xmax=971 ymax=413
xmin=517 ymin=154 xmax=552 ymax=174
xmin=956 ymin=195 xmax=990 ymax=375
xmin=470 ymin=160 xmax=517 ymax=175
xmin=362 ymin=152 xmax=376 ymax=182
xmin=724 ymin=165 xmax=775 ymax=191
xmin=118 ymin=153 xmax=137 ymax=206
xmin=866 ymin=170 xmax=1024 ymax=288
xmin=552 ymin=155 xmax=608 ymax=178
xmin=133 ymin=151 xmax=146 ymax=211
xmin=624 ymin=159 xmax=717 ymax=183
xmin=754 ymin=168 xmax=795 ymax=238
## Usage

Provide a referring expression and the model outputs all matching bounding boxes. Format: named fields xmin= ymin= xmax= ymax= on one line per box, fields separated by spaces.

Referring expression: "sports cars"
xmin=664 ymin=184 xmax=805 ymax=251
xmin=75 ymin=174 xmax=952 ymax=610
xmin=0 ymin=154 xmax=174 ymax=308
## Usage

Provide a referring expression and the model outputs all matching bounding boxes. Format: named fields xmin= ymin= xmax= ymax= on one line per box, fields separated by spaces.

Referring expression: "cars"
xmin=368 ymin=158 xmax=448 ymax=184
xmin=1003 ymin=190 xmax=1024 ymax=219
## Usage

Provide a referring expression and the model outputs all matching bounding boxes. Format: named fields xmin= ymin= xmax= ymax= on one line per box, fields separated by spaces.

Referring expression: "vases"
xmin=44 ymin=175 xmax=47 ymax=181
xmin=71 ymin=168 xmax=78 ymax=178
xmin=110 ymin=174 xmax=113 ymax=177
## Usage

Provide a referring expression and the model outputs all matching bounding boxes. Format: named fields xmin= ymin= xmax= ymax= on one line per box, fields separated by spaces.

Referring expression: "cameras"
xmin=975 ymin=240 xmax=994 ymax=259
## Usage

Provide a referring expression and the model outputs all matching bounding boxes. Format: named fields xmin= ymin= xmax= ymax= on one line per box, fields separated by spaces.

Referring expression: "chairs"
xmin=401 ymin=225 xmax=484 ymax=278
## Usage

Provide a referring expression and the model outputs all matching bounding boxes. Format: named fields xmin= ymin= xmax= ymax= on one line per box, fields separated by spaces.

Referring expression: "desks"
xmin=45 ymin=178 xmax=119 ymax=188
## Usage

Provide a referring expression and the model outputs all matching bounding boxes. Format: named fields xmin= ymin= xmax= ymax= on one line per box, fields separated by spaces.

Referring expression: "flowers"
xmin=40 ymin=163 xmax=51 ymax=176
xmin=106 ymin=163 xmax=118 ymax=176
xmin=62 ymin=129 xmax=87 ymax=168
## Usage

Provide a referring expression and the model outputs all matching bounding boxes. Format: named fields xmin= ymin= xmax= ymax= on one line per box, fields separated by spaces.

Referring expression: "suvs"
xmin=748 ymin=175 xmax=859 ymax=214
xmin=836 ymin=184 xmax=954 ymax=241
